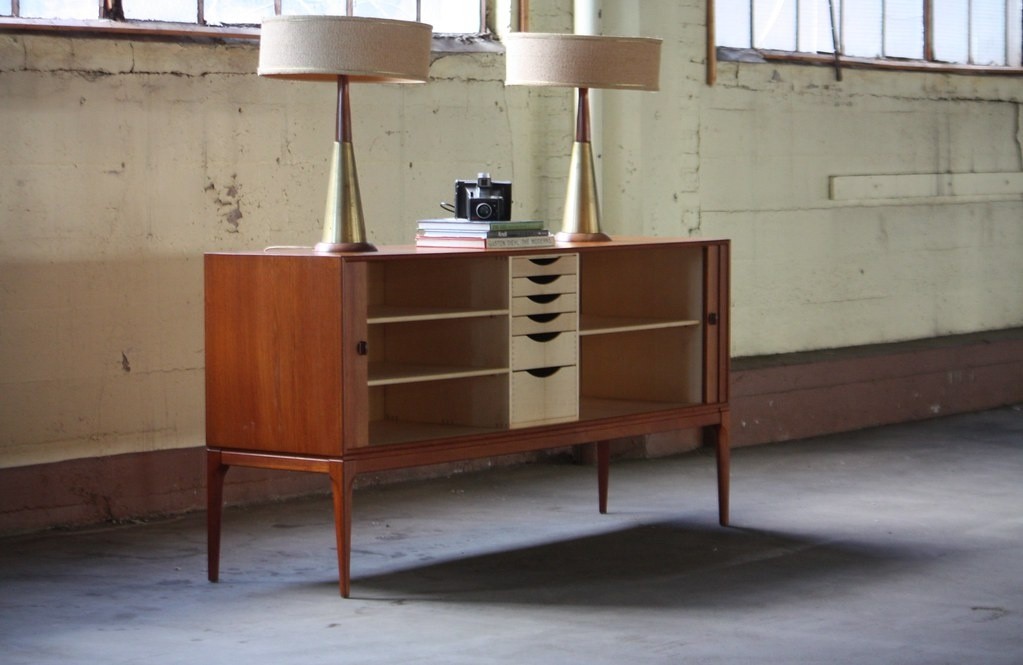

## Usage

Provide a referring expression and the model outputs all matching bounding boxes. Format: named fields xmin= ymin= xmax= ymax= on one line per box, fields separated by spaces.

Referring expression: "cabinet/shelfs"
xmin=201 ymin=238 xmax=732 ymax=599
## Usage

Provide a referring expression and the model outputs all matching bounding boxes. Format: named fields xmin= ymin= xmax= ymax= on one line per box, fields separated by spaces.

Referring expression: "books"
xmin=415 ymin=217 xmax=556 ymax=250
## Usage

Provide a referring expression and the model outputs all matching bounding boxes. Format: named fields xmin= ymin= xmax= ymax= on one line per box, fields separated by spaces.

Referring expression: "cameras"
xmin=454 ymin=172 xmax=512 ymax=221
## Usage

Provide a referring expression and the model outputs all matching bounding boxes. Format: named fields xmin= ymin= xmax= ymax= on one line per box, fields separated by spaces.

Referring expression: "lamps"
xmin=504 ymin=33 xmax=663 ymax=242
xmin=257 ymin=16 xmax=431 ymax=253
